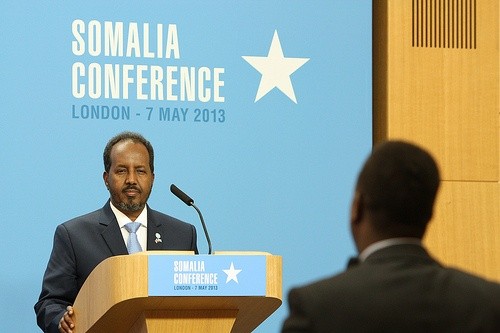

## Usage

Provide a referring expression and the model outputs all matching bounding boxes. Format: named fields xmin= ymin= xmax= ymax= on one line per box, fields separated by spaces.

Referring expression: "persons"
xmin=33 ymin=131 xmax=200 ymax=333
xmin=279 ymin=139 xmax=500 ymax=333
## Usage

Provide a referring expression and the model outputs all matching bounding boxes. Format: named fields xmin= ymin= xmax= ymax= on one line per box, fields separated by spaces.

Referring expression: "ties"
xmin=124 ymin=222 xmax=142 ymax=253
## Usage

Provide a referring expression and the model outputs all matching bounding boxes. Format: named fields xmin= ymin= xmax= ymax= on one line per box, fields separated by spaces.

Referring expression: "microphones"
xmin=170 ymin=184 xmax=212 ymax=254
